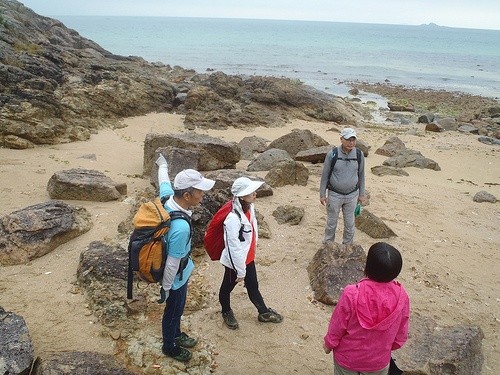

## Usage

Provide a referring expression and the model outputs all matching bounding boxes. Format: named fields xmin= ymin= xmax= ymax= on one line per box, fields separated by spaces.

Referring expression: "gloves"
xmin=155 ymin=153 xmax=167 ymax=166
xmin=156 ymin=287 xmax=170 ymax=303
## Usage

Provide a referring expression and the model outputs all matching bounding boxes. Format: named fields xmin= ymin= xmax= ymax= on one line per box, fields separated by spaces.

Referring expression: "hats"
xmin=231 ymin=177 xmax=264 ymax=197
xmin=174 ymin=169 xmax=215 ymax=191
xmin=340 ymin=128 xmax=357 ymax=139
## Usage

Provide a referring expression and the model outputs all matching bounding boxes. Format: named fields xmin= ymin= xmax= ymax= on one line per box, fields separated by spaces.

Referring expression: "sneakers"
xmin=222 ymin=310 xmax=239 ymax=329
xmin=171 ymin=348 xmax=191 ymax=361
xmin=174 ymin=332 xmax=198 ymax=348
xmin=258 ymin=308 xmax=283 ymax=323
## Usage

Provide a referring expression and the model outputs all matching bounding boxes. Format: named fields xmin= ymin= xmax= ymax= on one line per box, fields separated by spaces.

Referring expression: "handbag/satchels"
xmin=387 ymin=357 xmax=403 ymax=375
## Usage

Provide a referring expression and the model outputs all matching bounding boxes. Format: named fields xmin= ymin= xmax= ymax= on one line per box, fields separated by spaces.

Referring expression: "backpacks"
xmin=128 ymin=195 xmax=192 ymax=283
xmin=204 ymin=199 xmax=242 ymax=261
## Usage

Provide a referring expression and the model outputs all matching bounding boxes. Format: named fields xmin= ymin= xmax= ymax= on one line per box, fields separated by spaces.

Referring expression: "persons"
xmin=318 ymin=130 xmax=369 ymax=247
xmin=147 ymin=153 xmax=216 ymax=362
xmin=218 ymin=177 xmax=285 ymax=329
xmin=323 ymin=242 xmax=410 ymax=375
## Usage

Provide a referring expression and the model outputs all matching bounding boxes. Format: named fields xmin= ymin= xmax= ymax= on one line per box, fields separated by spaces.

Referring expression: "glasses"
xmin=343 ymin=137 xmax=355 ymax=141
